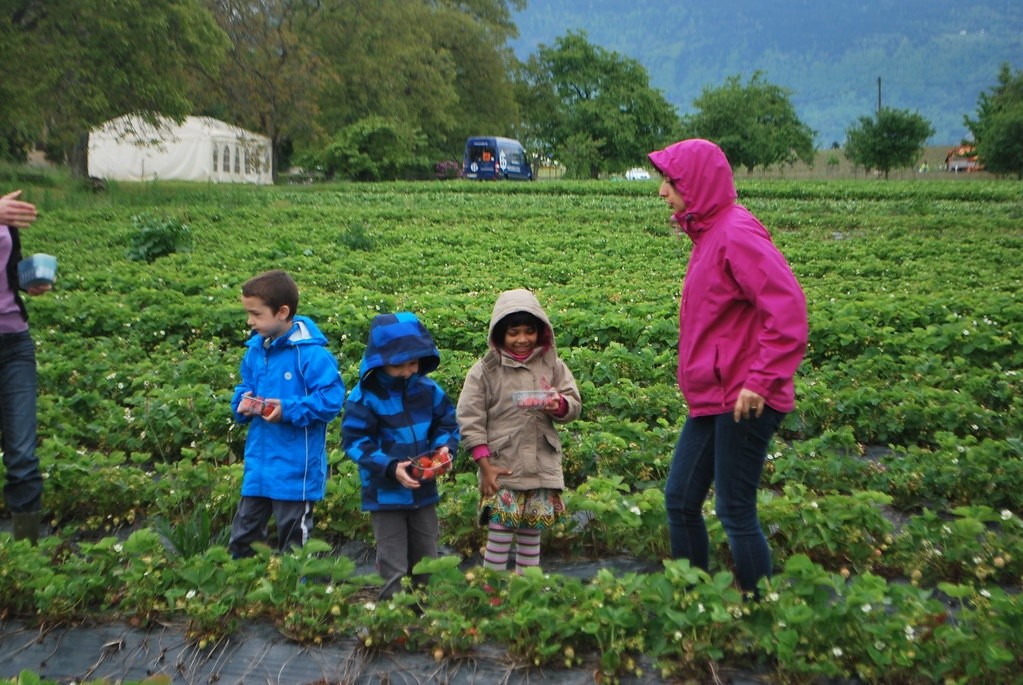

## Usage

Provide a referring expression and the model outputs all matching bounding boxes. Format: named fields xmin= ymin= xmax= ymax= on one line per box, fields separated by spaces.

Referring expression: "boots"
xmin=10 ymin=510 xmax=41 ymax=546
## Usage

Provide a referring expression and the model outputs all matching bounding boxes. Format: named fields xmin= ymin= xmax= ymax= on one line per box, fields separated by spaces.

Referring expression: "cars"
xmin=624 ymin=165 xmax=650 ymax=181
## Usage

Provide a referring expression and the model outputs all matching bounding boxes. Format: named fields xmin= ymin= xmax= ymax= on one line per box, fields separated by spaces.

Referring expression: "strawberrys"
xmin=3 ymin=512 xmax=1014 ymax=685
xmin=518 ymin=396 xmax=550 ymax=407
xmin=240 ymin=395 xmax=274 ymax=416
xmin=412 ymin=454 xmax=450 ymax=480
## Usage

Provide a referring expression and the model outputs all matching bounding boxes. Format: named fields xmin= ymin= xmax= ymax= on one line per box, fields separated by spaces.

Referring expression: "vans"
xmin=461 ymin=136 xmax=534 ymax=183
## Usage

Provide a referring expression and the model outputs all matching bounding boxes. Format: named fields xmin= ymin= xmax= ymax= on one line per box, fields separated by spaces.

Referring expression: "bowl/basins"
xmin=17 ymin=253 xmax=57 ymax=289
xmin=511 ymin=390 xmax=554 ymax=413
xmin=236 ymin=394 xmax=275 ymax=416
xmin=410 ymin=449 xmax=453 ymax=481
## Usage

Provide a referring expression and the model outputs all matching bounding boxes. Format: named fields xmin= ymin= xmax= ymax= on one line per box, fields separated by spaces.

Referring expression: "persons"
xmin=455 ymin=289 xmax=583 ymax=578
xmin=342 ymin=311 xmax=459 ymax=614
xmin=648 ymin=139 xmax=809 ymax=601
xmin=226 ymin=270 xmax=346 ymax=558
xmin=0 ymin=190 xmax=51 ymax=545
xmin=919 ymin=161 xmax=929 ymax=175
